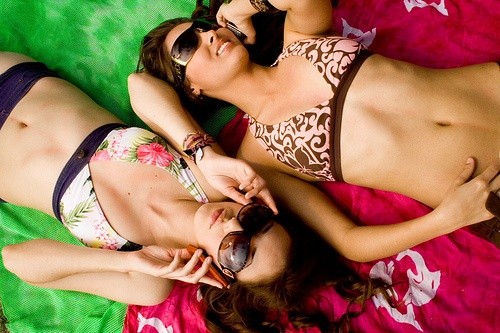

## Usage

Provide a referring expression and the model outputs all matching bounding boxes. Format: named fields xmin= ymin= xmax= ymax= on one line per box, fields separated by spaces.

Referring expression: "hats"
xmin=170 ymin=21 xmax=219 ymax=88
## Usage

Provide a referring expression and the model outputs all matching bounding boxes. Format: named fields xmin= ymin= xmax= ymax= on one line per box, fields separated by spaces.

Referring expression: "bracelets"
xmin=250 ymin=0 xmax=285 ymax=16
xmin=180 ymin=130 xmax=214 ymax=164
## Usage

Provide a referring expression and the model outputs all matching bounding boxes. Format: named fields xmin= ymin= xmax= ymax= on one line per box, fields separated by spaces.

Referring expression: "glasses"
xmin=218 ymin=202 xmax=274 ymax=284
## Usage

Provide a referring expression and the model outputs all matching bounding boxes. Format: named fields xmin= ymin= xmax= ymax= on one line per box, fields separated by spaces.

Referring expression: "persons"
xmin=137 ymin=1 xmax=499 ymax=265
xmin=0 ymin=50 xmax=328 ymax=307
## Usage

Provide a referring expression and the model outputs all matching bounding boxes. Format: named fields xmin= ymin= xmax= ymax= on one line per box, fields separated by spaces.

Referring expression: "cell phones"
xmin=225 ymin=21 xmax=244 ymax=40
xmin=188 ymin=242 xmax=231 ymax=290
